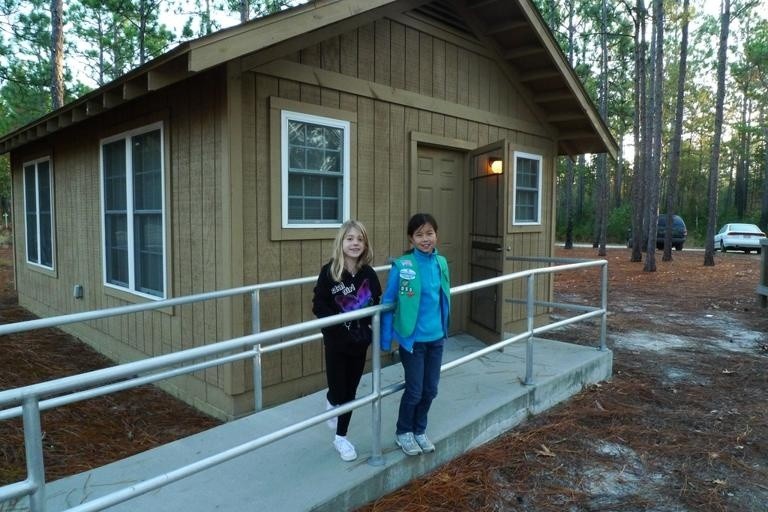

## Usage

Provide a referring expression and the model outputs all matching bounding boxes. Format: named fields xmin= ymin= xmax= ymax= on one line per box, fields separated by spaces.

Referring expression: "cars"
xmin=714 ymin=223 xmax=766 ymax=253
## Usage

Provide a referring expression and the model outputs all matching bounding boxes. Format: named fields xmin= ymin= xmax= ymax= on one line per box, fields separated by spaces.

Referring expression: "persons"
xmin=379 ymin=213 xmax=452 ymax=456
xmin=310 ymin=220 xmax=382 ymax=461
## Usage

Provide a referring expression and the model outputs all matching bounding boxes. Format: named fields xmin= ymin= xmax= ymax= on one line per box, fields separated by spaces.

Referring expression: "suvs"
xmin=627 ymin=214 xmax=688 ymax=250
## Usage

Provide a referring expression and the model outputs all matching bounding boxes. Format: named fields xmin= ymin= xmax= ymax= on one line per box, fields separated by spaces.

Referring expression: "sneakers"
xmin=334 ymin=435 xmax=357 ymax=461
xmin=327 ymin=400 xmax=338 ymax=429
xmin=396 ymin=432 xmax=435 ymax=456
